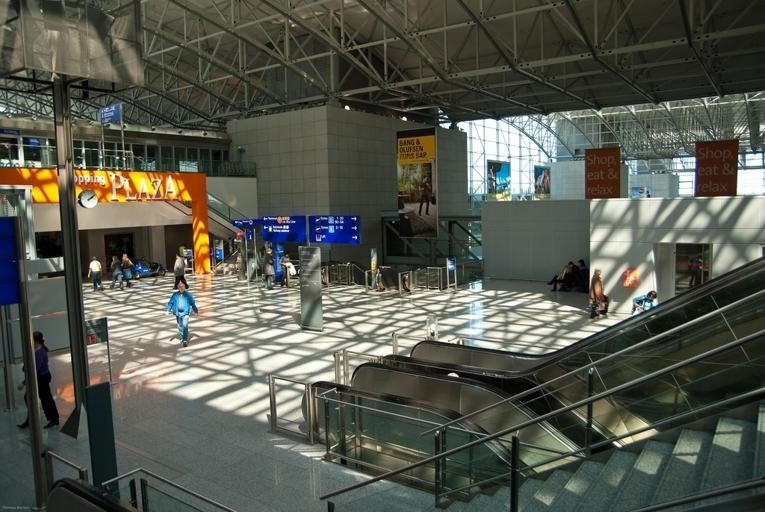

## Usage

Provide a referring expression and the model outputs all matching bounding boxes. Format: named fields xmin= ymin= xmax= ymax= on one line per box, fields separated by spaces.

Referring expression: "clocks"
xmin=79 ymin=189 xmax=98 ymax=210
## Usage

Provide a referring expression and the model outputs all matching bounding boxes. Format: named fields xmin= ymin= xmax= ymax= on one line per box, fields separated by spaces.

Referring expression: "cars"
xmin=131 ymin=259 xmax=165 ymax=280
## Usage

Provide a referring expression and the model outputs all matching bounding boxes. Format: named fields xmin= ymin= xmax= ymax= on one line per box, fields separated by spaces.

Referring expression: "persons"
xmin=87 ymin=255 xmax=103 ymax=291
xmin=578 ymin=259 xmax=586 ymax=271
xmin=111 ymin=256 xmax=123 ymax=290
xmin=173 ymin=251 xmax=189 ymax=290
xmin=589 ymin=269 xmax=604 ymax=318
xmin=568 ymin=261 xmax=580 ymax=272
xmin=264 ymin=247 xmax=275 ymax=290
xmin=165 ymin=283 xmax=200 ymax=346
xmin=17 ymin=331 xmax=59 ymax=430
xmin=418 ymin=176 xmax=431 ymax=216
xmin=279 ymin=253 xmax=292 ymax=288
xmin=631 ymin=291 xmax=658 ymax=316
xmin=122 ymin=254 xmax=134 ymax=287
xmin=548 ymin=265 xmax=573 ymax=290
